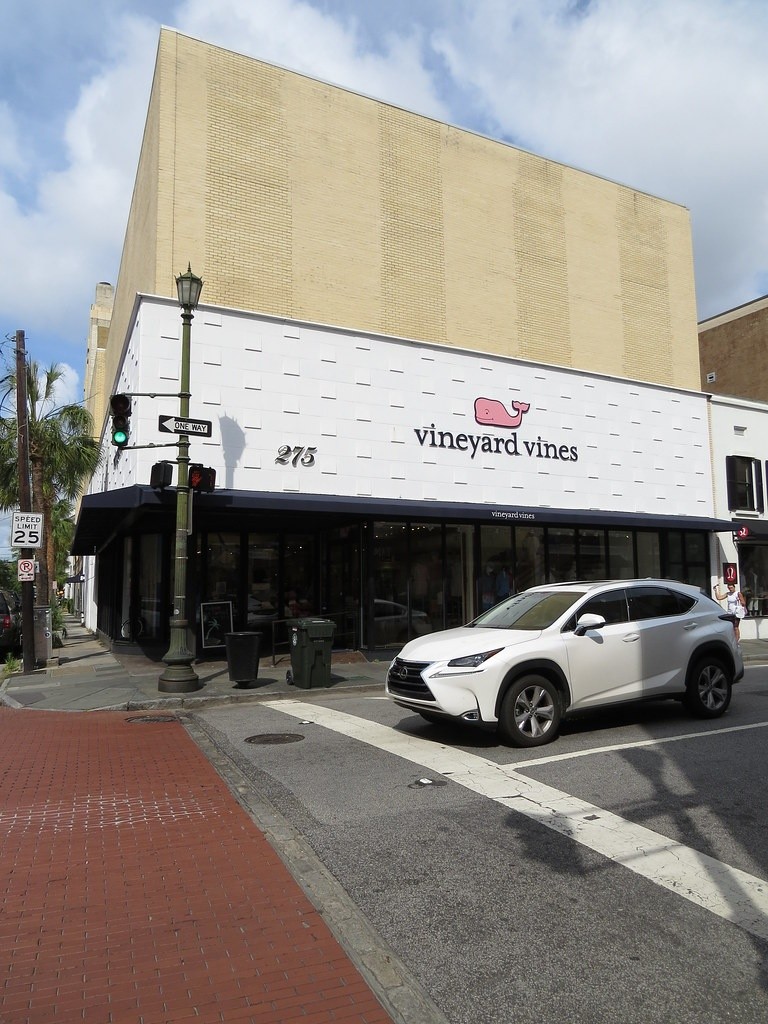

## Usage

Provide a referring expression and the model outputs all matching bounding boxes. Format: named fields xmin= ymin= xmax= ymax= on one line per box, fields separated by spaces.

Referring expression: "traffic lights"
xmin=109 ymin=393 xmax=132 ymax=448
xmin=188 ymin=464 xmax=207 ymax=490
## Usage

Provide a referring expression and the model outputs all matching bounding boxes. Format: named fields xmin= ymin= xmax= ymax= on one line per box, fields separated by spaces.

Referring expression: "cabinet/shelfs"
xmin=201 ymin=526 xmax=322 ymax=624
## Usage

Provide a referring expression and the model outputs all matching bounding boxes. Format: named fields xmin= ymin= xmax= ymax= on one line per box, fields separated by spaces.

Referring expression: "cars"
xmin=0 ymin=590 xmax=22 ymax=656
xmin=373 ymin=597 xmax=433 ymax=649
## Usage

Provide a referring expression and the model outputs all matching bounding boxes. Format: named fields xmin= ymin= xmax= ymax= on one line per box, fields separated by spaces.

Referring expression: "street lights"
xmin=157 ymin=260 xmax=207 ymax=693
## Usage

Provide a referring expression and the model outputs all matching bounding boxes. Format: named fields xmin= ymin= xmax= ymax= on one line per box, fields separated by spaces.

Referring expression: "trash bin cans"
xmin=285 ymin=617 xmax=338 ymax=690
xmin=224 ymin=632 xmax=261 ymax=688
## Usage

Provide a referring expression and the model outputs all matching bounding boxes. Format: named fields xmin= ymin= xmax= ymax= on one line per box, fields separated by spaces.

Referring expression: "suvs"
xmin=385 ymin=576 xmax=744 ymax=748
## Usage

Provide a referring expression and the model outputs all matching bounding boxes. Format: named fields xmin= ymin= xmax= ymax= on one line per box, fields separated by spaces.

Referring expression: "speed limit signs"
xmin=11 ymin=511 xmax=43 ymax=549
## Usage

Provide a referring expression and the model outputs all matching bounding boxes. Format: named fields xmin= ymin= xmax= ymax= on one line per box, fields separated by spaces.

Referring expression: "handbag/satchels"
xmin=735 ymin=598 xmax=746 ymax=619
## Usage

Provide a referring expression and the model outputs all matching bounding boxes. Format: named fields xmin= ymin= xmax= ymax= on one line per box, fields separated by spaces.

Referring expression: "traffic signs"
xmin=158 ymin=414 xmax=212 ymax=438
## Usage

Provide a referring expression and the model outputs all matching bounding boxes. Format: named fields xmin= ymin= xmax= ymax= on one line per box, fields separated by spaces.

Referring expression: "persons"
xmin=480 ymin=555 xmax=512 ymax=606
xmin=741 ymin=584 xmax=768 ymax=616
xmin=714 ymin=583 xmax=745 ymax=647
